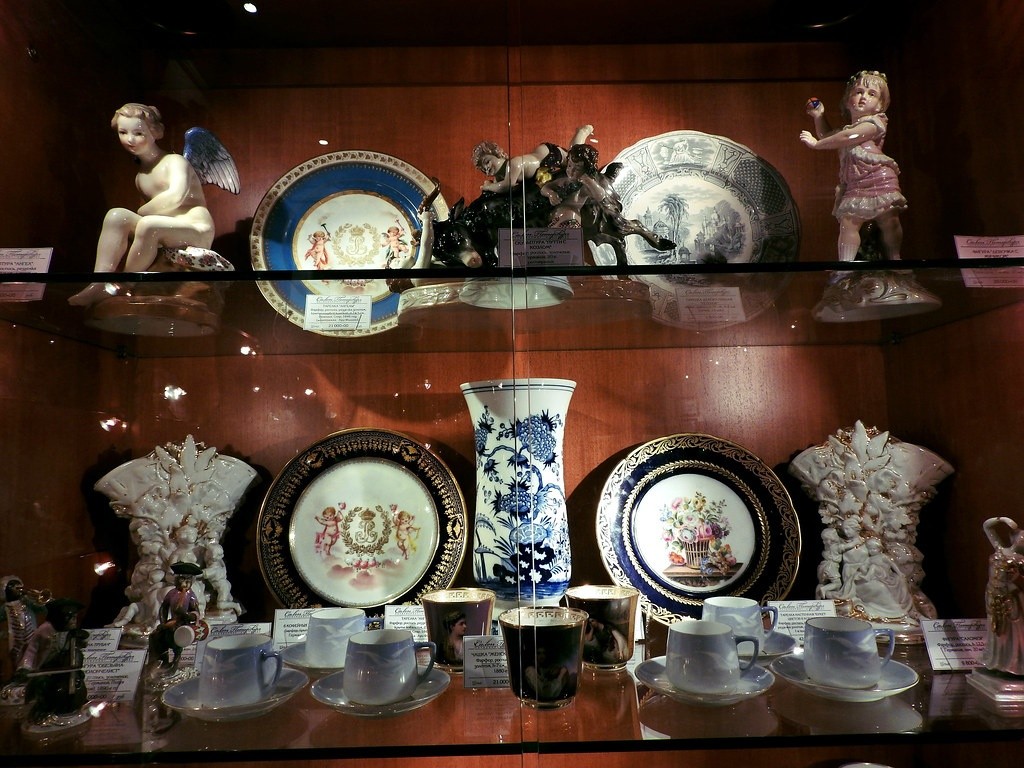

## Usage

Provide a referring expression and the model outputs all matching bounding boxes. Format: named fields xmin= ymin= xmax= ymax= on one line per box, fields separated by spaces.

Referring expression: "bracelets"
xmin=997 ymin=517 xmax=1001 ymax=524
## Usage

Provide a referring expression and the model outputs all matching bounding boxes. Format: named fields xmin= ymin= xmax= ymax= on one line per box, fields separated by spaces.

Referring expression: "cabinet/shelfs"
xmin=0 ymin=0 xmax=1023 ymax=768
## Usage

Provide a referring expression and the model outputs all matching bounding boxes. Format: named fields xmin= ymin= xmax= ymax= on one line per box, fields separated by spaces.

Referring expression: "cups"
xmin=665 ymin=620 xmax=760 ymax=692
xmin=305 ymin=607 xmax=383 ymax=666
xmin=499 ymin=606 xmax=589 ymax=710
xmin=564 ymin=585 xmax=639 ymax=671
xmin=422 ymin=588 xmax=495 ymax=674
xmin=804 ymin=618 xmax=895 ymax=689
xmin=343 ymin=630 xmax=436 ymax=704
xmin=703 ymin=596 xmax=778 ymax=652
xmin=201 ymin=633 xmax=281 ymax=707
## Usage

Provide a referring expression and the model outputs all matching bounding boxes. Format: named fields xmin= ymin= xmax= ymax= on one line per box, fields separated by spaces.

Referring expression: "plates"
xmin=250 ymin=151 xmax=451 ymax=339
xmin=595 ymin=432 xmax=800 ymax=632
xmin=309 ymin=666 xmax=450 ymax=717
xmin=278 ymin=642 xmax=346 ymax=674
xmin=256 ymin=427 xmax=470 ymax=632
xmin=161 ymin=668 xmax=309 ymax=722
xmin=768 ymin=653 xmax=919 ymax=702
xmin=588 ymin=130 xmax=797 ymax=331
xmin=635 ymin=660 xmax=775 ymax=706
xmin=736 ymin=629 xmax=798 ymax=667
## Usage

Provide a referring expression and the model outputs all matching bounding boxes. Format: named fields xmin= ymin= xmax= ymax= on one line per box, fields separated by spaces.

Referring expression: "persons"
xmin=67 ymin=103 xmax=215 ymax=306
xmin=471 ymin=125 xmax=606 ymax=226
xmin=387 ymin=207 xmax=498 ymax=292
xmin=105 ymin=524 xmax=242 ymax=676
xmin=982 ymin=517 xmax=1024 ymax=675
xmin=799 ymin=70 xmax=915 ymax=275
xmin=817 ymin=519 xmax=912 ymax=616
xmin=0 ymin=576 xmax=77 ymax=726
